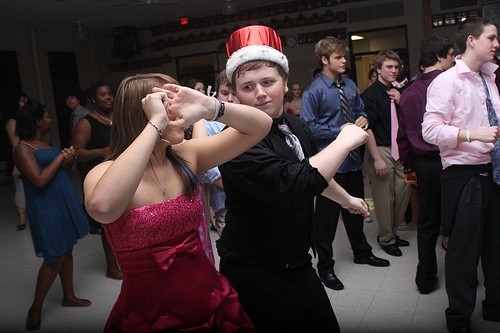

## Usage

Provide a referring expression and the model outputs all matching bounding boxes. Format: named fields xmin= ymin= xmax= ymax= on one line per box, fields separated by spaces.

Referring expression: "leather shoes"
xmin=354 ymin=252 xmax=390 ymax=266
xmin=381 ymin=244 xmax=402 ymax=256
xmin=377 ymin=234 xmax=409 ymax=246
xmin=320 ymin=272 xmax=344 ymax=290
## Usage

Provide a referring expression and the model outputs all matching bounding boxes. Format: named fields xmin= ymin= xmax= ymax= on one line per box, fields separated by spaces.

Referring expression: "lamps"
xmin=221 ymin=0 xmax=239 ymax=16
xmin=72 ymin=20 xmax=89 ymax=44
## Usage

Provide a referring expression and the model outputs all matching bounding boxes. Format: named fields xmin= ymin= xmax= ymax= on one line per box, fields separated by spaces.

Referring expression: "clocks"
xmin=285 ymin=36 xmax=297 ymax=48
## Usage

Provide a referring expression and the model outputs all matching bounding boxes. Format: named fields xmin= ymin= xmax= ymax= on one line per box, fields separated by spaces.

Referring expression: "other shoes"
xmin=442 ymin=240 xmax=448 ymax=251
xmin=17 ymin=223 xmax=25 ymax=230
xmin=448 ymin=327 xmax=472 ymax=333
xmin=364 ymin=216 xmax=371 ymax=222
xmin=418 ymin=278 xmax=440 ymax=294
xmin=399 ymin=225 xmax=418 ymax=231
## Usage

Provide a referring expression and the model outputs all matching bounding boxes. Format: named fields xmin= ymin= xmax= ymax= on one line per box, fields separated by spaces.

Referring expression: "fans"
xmin=114 ymin=0 xmax=178 ymax=9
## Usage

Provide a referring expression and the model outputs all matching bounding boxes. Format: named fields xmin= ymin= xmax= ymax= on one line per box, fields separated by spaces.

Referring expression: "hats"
xmin=226 ymin=25 xmax=289 ymax=84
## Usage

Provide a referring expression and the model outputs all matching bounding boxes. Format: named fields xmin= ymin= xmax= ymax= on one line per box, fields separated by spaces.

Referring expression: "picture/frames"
xmin=479 ymin=2 xmax=500 ymax=60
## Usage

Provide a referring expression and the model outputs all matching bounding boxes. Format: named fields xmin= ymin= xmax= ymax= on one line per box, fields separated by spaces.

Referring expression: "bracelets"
xmin=146 ymin=120 xmax=164 ymax=139
xmin=206 ymin=96 xmax=220 ymax=122
xmin=465 ymin=128 xmax=471 ymax=143
xmin=214 ymin=100 xmax=225 ymax=121
xmin=84 ymin=73 xmax=273 ymax=333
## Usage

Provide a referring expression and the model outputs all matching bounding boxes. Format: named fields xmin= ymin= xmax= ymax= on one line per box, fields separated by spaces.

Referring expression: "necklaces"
xmin=92 ymin=107 xmax=114 ymax=126
xmin=145 ymin=154 xmax=168 ymax=195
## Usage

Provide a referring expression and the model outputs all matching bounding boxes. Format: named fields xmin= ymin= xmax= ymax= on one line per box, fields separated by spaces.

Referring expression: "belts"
xmin=442 ymin=165 xmax=492 ymax=174
xmin=376 ymin=143 xmax=391 ymax=147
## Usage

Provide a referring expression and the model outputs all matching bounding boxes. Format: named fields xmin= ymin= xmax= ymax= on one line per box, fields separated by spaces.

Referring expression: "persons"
xmin=491 ymin=56 xmax=500 ymax=93
xmin=421 ymin=17 xmax=500 ymax=333
xmin=283 ymin=81 xmax=302 ymax=116
xmin=65 ymin=90 xmax=92 ymax=138
xmin=4 ymin=90 xmax=30 ymax=231
xmin=73 ymin=80 xmax=123 ymax=280
xmin=312 ymin=56 xmax=428 ymax=91
xmin=397 ymin=32 xmax=480 ymax=293
xmin=360 ymin=50 xmax=412 ymax=256
xmin=182 ymin=68 xmax=240 ymax=237
xmin=300 ymin=37 xmax=390 ymax=290
xmin=14 ymin=100 xmax=92 ymax=332
xmin=218 ymin=26 xmax=370 ymax=333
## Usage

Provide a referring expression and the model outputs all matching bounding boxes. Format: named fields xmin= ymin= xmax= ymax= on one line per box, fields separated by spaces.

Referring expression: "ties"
xmin=278 ymin=118 xmax=305 ymax=162
xmin=391 ymin=99 xmax=399 ymax=160
xmin=334 ymin=81 xmax=360 ymax=161
xmin=479 ymin=70 xmax=500 ymax=184
